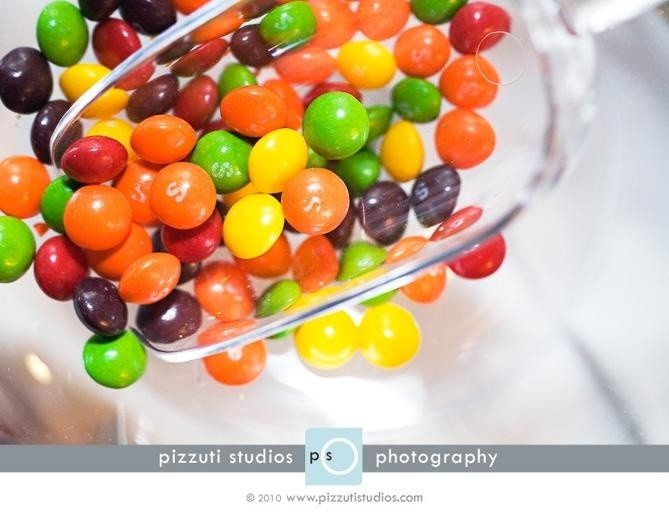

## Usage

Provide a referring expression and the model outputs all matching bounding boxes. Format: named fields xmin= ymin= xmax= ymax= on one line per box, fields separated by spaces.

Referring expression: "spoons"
xmin=48 ymin=0 xmax=669 ymax=362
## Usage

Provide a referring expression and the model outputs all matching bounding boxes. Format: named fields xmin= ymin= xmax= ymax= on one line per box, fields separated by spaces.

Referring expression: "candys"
xmin=0 ymin=0 xmax=512 ymax=391
